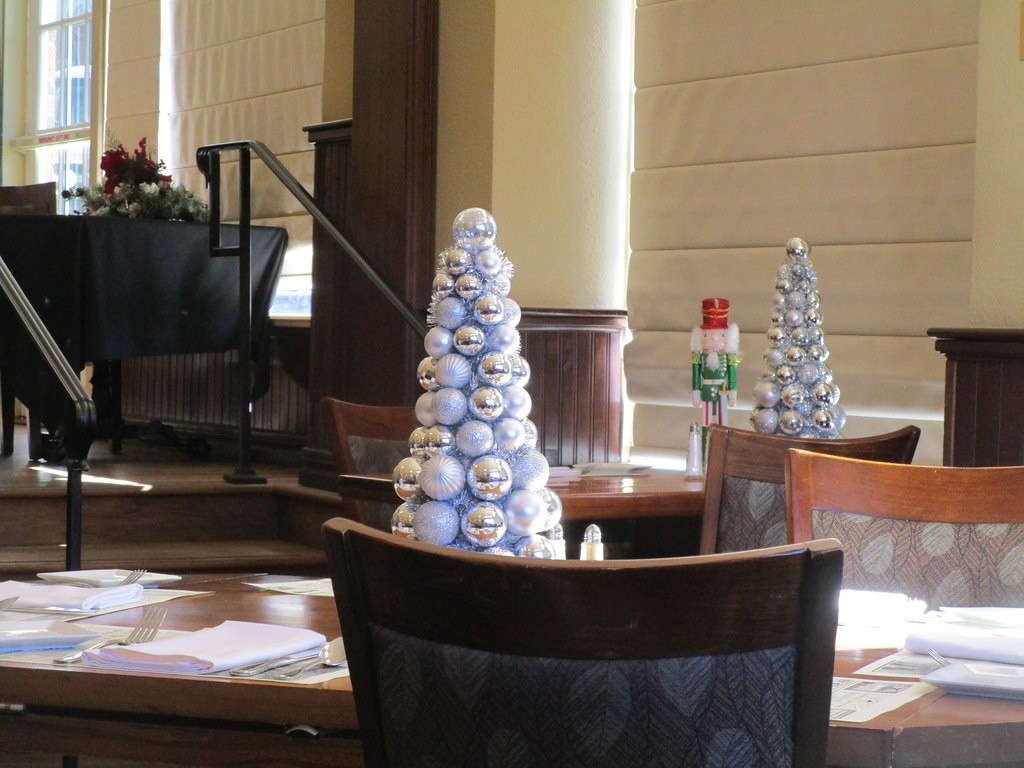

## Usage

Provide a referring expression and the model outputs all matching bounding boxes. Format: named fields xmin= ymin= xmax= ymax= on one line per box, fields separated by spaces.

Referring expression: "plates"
xmin=37 ymin=569 xmax=182 ymax=588
xmin=919 ymin=662 xmax=1024 ymax=700
xmin=0 ymin=619 xmax=99 ymax=652
xmin=572 ymin=463 xmax=651 ymax=472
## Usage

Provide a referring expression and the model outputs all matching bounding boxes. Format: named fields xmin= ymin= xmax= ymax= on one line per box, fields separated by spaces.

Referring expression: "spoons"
xmin=282 ymin=636 xmax=347 ymax=678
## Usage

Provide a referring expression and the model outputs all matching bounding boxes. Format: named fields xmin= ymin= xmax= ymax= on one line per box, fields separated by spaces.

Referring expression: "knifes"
xmin=231 ymin=651 xmax=319 ymax=676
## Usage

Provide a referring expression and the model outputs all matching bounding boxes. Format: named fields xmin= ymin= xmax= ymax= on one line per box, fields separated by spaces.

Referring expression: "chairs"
xmin=319 ymin=395 xmax=425 ymax=534
xmin=0 ymin=181 xmax=57 ymax=214
xmin=320 ymin=517 xmax=845 ymax=768
xmin=700 ymin=422 xmax=921 ymax=556
xmin=786 ymin=448 xmax=1024 ymax=611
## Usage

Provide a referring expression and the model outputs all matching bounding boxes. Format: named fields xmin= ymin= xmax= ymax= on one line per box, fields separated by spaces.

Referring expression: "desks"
xmin=339 ymin=462 xmax=706 ymax=560
xmin=1 ymin=213 xmax=288 ymax=461
xmin=0 ymin=571 xmax=1023 ymax=768
xmin=927 ymin=327 xmax=1024 ymax=467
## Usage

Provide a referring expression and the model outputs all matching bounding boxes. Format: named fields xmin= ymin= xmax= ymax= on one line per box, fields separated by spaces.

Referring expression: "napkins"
xmin=81 ymin=620 xmax=327 ymax=675
xmin=905 ymin=621 xmax=1023 ymax=666
xmin=0 ymin=580 xmax=144 ymax=612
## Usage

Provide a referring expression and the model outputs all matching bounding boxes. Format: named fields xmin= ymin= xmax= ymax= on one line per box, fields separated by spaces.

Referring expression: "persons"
xmin=690 ymin=298 xmax=739 ymax=476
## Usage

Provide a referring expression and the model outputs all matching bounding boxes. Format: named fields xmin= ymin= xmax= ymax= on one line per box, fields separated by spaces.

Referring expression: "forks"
xmin=53 ymin=606 xmax=168 ymax=663
xmin=927 ymin=648 xmax=964 ymax=670
xmin=71 ymin=569 xmax=147 ymax=589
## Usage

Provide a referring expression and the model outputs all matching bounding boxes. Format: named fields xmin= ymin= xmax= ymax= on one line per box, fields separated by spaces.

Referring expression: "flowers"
xmin=62 ymin=132 xmax=210 ymax=224
